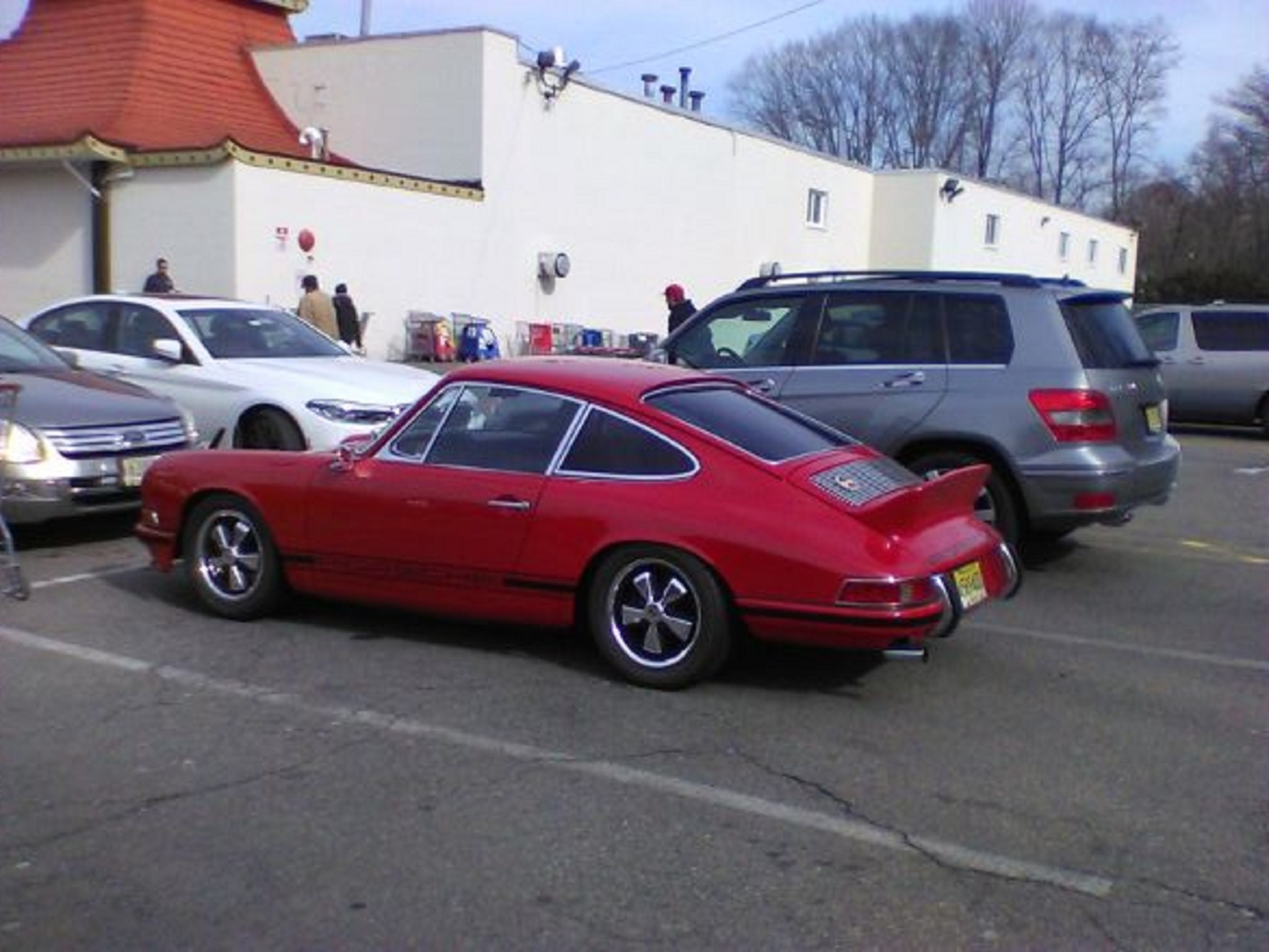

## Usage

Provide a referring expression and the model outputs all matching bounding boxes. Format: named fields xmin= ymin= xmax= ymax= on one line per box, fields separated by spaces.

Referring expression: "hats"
xmin=662 ymin=284 xmax=684 ymax=302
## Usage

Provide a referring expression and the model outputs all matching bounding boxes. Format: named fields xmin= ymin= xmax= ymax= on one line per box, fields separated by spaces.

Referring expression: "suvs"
xmin=485 ymin=270 xmax=1183 ymax=572
xmin=1129 ymin=300 xmax=1269 ymax=440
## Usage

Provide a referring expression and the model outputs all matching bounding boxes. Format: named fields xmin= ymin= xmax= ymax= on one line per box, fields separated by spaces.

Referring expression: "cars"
xmin=0 ymin=313 xmax=199 ymax=547
xmin=133 ymin=355 xmax=1018 ymax=692
xmin=13 ymin=293 xmax=487 ymax=462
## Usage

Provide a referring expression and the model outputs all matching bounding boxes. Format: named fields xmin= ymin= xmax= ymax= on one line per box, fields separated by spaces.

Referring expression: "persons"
xmin=297 ymin=275 xmax=362 ymax=349
xmin=143 ymin=259 xmax=174 ymax=292
xmin=662 ymin=285 xmax=712 ymax=364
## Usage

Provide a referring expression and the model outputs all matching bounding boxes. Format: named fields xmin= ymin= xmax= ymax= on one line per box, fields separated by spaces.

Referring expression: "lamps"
xmin=537 ymin=51 xmax=581 ymax=98
xmin=940 ymin=179 xmax=964 ymax=203
